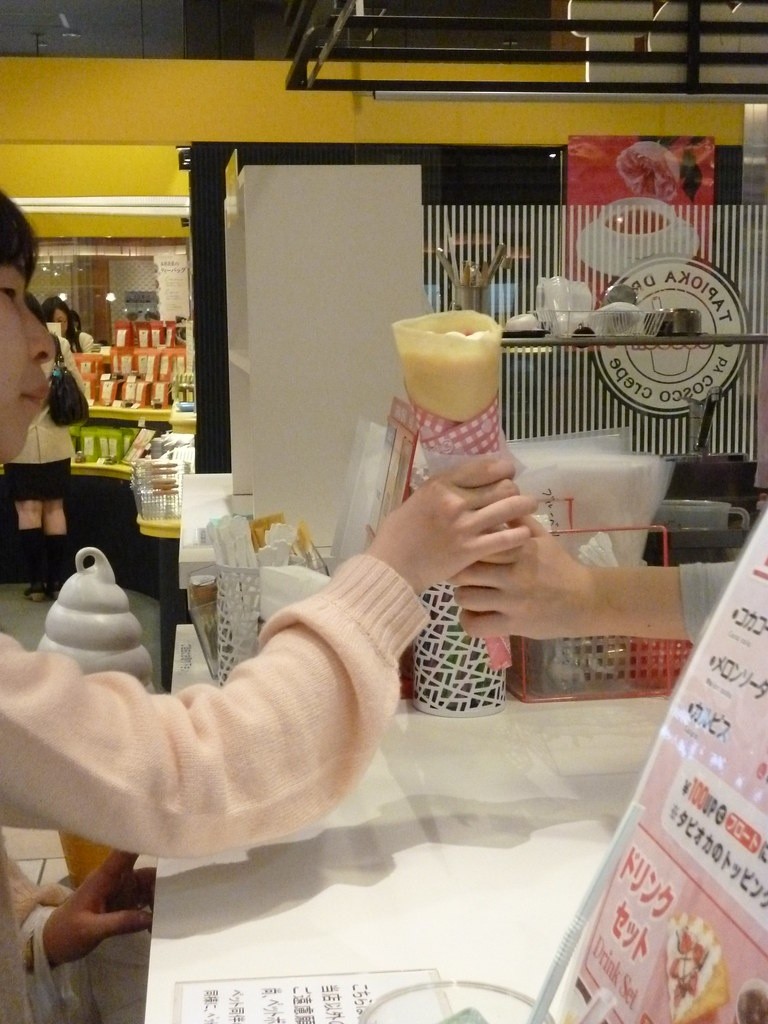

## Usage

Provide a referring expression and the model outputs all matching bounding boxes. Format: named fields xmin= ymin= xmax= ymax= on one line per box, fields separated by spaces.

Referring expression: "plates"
xmin=575 ymin=214 xmax=701 ymax=277
xmin=501 ymin=328 xmax=551 ymax=339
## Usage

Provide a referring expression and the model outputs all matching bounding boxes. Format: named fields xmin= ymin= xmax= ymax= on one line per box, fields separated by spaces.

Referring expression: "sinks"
xmin=665 ymin=459 xmax=759 ymax=499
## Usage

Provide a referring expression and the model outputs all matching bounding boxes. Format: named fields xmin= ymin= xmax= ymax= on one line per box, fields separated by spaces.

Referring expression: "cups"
xmin=454 ymin=285 xmax=492 ymax=318
xmin=652 ymin=495 xmax=752 ymax=534
xmin=600 ymin=196 xmax=677 ymax=259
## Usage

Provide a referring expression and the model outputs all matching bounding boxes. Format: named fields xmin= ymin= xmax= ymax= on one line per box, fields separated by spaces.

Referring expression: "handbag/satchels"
xmin=20 ymin=906 xmax=99 ymax=1024
xmin=49 ymin=333 xmax=90 ymax=426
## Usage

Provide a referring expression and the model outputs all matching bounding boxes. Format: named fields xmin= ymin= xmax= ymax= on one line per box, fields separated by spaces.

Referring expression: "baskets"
xmin=509 ymin=525 xmax=692 ymax=703
xmin=216 ymin=559 xmax=307 ymax=693
xmin=411 ymin=578 xmax=508 ymax=718
xmin=130 ymin=457 xmax=186 ymax=519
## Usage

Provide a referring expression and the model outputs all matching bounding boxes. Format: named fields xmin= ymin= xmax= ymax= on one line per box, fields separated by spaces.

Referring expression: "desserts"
xmin=665 ymin=911 xmax=728 ymax=1024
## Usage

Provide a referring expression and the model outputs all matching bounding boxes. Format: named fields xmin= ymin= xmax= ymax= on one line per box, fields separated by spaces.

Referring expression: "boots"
xmin=45 ymin=535 xmax=69 ymax=600
xmin=18 ymin=527 xmax=50 ymax=601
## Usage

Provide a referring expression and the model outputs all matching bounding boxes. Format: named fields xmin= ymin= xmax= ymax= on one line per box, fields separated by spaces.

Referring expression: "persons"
xmin=0 ymin=190 xmax=535 ymax=1024
xmin=40 ymin=297 xmax=93 ymax=354
xmin=452 ymin=515 xmax=740 ymax=649
xmin=4 ymin=292 xmax=74 ymax=602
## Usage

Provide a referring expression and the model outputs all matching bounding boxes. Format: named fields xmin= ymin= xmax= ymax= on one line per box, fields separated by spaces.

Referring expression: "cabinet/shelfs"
xmin=0 ymin=374 xmax=197 ymax=600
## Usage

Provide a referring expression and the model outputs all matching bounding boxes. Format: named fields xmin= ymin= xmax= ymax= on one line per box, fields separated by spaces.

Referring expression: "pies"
xmin=392 ymin=310 xmax=502 ymax=422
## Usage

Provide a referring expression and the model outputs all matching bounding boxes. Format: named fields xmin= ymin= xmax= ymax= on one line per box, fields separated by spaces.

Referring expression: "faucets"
xmin=676 ymin=385 xmax=723 ymax=457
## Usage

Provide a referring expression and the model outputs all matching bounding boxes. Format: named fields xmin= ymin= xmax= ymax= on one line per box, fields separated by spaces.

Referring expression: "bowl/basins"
xmin=653 ymin=306 xmax=703 ymax=334
